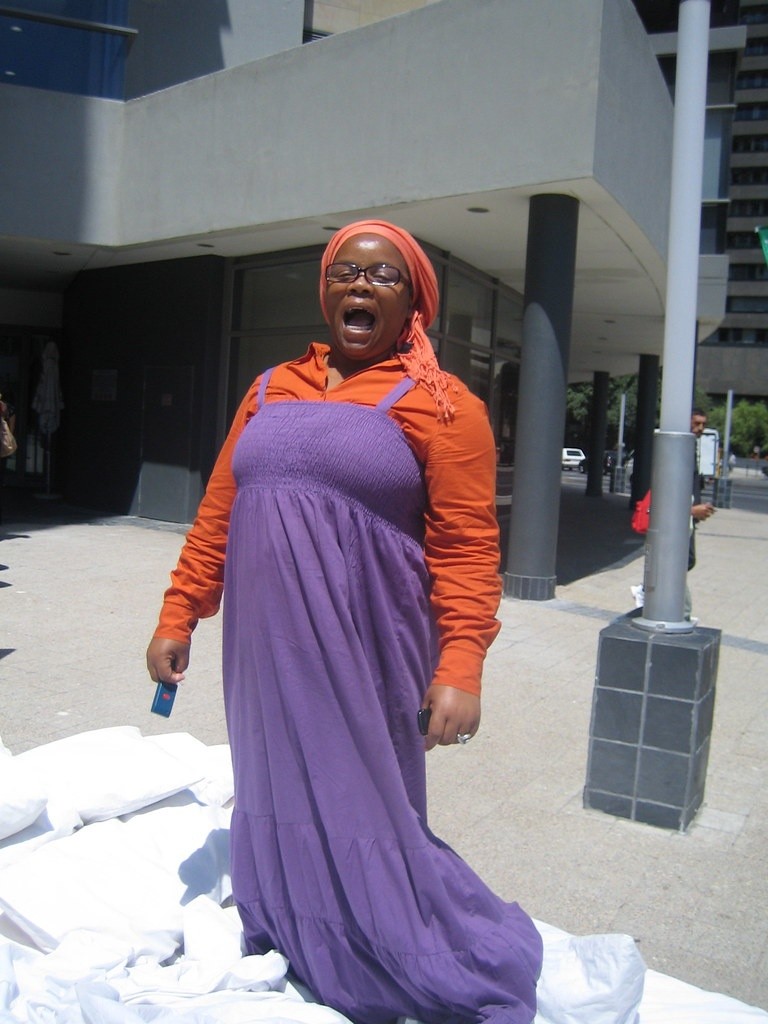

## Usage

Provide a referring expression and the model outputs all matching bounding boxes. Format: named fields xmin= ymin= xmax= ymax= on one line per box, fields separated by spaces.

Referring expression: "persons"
xmin=729 ymin=453 xmax=736 ymax=473
xmin=0 ymin=397 xmax=16 ymax=498
xmin=629 ymin=407 xmax=715 ymax=620
xmin=146 ymin=217 xmax=544 ymax=1024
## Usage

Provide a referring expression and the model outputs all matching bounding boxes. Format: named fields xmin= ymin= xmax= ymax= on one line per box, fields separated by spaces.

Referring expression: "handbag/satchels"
xmin=0 ymin=417 xmax=18 ymax=458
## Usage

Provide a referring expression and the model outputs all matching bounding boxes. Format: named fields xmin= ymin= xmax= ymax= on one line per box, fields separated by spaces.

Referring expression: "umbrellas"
xmin=31 ymin=341 xmax=63 ymax=500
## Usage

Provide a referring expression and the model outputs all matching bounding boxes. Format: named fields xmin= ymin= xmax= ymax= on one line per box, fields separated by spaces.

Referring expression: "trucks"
xmin=696 ymin=426 xmax=719 ymax=490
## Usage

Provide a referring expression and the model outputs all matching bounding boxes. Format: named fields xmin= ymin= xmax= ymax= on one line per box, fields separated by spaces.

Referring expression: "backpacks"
xmin=631 ymin=490 xmax=651 ymax=534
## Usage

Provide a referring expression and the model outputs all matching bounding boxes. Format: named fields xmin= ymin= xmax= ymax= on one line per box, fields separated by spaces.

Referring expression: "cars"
xmin=624 ymin=457 xmax=635 ymax=485
xmin=579 ymin=451 xmax=626 ymax=474
xmin=562 ymin=448 xmax=586 ymax=471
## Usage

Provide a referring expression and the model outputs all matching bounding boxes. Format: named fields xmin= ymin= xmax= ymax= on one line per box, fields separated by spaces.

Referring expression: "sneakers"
xmin=630 ymin=584 xmax=645 ymax=608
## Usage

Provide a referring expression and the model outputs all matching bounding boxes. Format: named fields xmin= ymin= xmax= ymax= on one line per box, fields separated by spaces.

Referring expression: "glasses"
xmin=325 ymin=262 xmax=412 ymax=288
